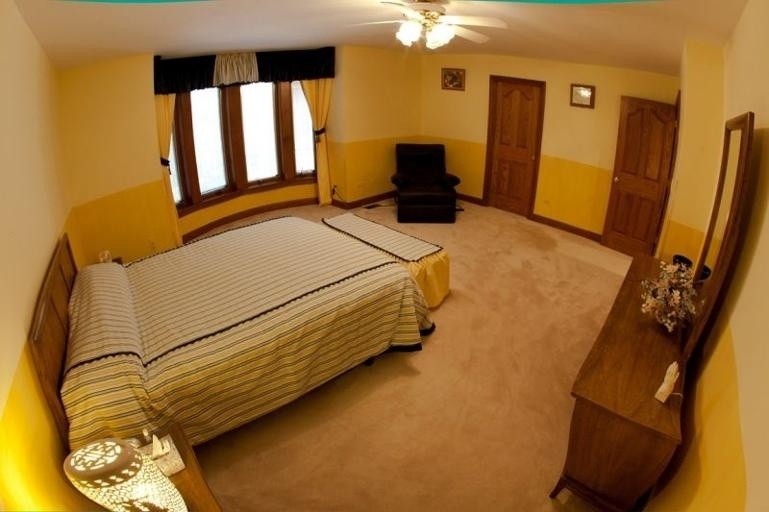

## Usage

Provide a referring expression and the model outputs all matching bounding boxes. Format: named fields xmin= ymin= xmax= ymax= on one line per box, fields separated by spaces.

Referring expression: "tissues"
xmin=136 ymin=433 xmax=186 ymax=477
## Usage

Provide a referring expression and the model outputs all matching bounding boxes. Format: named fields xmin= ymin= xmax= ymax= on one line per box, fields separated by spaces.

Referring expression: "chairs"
xmin=392 ymin=144 xmax=461 ymax=223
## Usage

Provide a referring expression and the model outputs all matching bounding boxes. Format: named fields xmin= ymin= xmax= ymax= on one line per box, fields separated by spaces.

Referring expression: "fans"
xmin=346 ymin=1 xmax=507 ymax=45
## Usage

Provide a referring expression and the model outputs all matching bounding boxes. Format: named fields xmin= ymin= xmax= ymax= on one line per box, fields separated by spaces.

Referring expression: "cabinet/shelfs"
xmin=551 ymin=261 xmax=683 ymax=509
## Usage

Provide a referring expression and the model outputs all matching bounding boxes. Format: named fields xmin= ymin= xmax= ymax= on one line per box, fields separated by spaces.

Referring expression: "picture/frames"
xmin=441 ymin=68 xmax=465 ymax=91
xmin=570 ymin=84 xmax=595 ymax=108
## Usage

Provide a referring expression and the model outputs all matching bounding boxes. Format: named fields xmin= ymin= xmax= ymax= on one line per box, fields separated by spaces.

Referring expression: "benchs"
xmin=322 ymin=210 xmax=449 ymax=310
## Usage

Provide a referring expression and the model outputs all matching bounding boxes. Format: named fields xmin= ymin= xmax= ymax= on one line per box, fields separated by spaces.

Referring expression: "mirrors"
xmin=681 ymin=110 xmax=755 ymax=365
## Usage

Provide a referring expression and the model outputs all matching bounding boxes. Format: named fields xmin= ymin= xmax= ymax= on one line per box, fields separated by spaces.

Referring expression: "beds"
xmin=28 ymin=214 xmax=436 ymax=453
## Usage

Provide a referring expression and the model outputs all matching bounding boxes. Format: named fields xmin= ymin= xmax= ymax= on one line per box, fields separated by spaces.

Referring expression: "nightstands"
xmin=135 ymin=423 xmax=223 ymax=512
xmin=111 ymin=258 xmax=122 ymax=263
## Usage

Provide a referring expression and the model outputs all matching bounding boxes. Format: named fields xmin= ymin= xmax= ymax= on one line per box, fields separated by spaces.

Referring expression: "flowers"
xmin=638 ymin=260 xmax=695 ymax=334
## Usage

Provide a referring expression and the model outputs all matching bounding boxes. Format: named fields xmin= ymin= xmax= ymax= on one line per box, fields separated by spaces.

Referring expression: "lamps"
xmin=395 ymin=22 xmax=455 ymax=50
xmin=63 ymin=436 xmax=189 ymax=512
xmin=96 ymin=250 xmax=111 ymax=262
